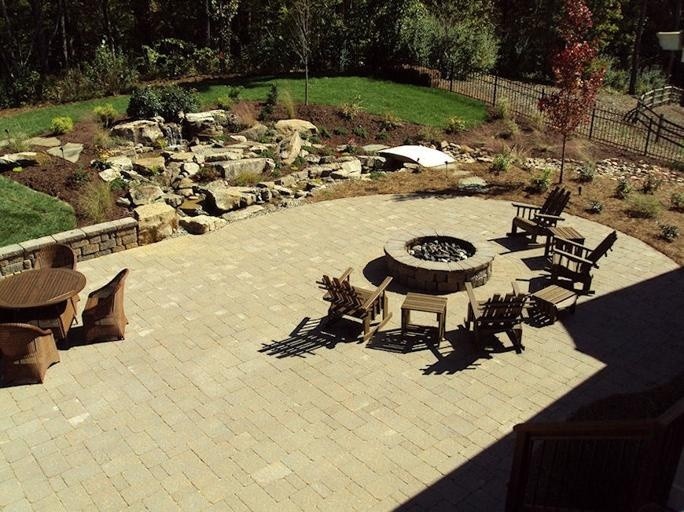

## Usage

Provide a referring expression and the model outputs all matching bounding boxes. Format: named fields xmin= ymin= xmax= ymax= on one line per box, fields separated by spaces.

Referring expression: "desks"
xmin=400 ymin=292 xmax=448 ymax=347
xmin=0 ymin=268 xmax=86 ymax=340
xmin=528 ymin=285 xmax=578 ymax=325
xmin=543 ymin=226 xmax=585 ymax=261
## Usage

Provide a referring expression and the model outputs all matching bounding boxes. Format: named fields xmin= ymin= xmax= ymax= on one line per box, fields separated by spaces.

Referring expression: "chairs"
xmin=34 ymin=245 xmax=80 ymax=303
xmin=315 ymin=267 xmax=393 ymax=344
xmin=462 ymin=280 xmax=532 ymax=355
xmin=506 ymin=186 xmax=572 ymax=248
xmin=81 ymin=268 xmax=130 ymax=345
xmin=0 ymin=322 xmax=60 ymax=384
xmin=550 ymin=231 xmax=617 ymax=295
